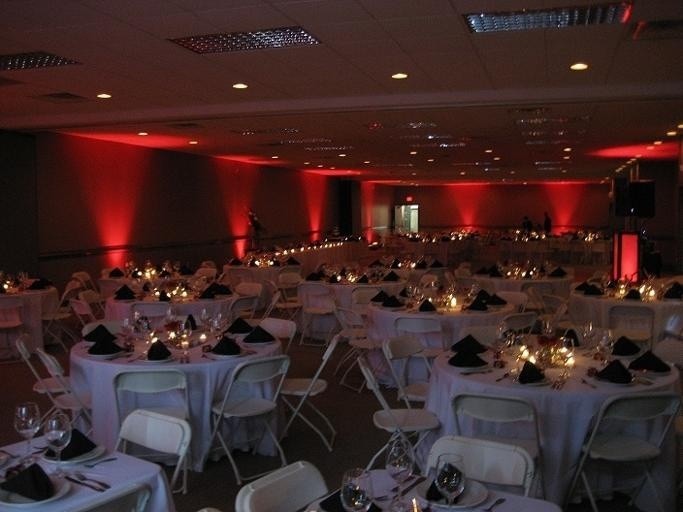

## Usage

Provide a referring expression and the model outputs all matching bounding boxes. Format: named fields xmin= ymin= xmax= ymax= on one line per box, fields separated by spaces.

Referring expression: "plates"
xmin=574 ymin=275 xmax=683 ymax=301
xmin=305 ymin=266 xmax=400 ymax=285
xmin=0 ymin=451 xmax=9 ymax=465
xmin=0 ymin=473 xmax=71 ymax=507
xmin=371 ymin=281 xmax=507 ymax=314
xmin=445 ymin=321 xmax=672 ymax=392
xmin=83 ymin=307 xmax=277 ymax=363
xmin=41 ymin=442 xmax=106 ymax=464
xmin=109 ymin=265 xmax=232 ymax=304
xmin=415 ymin=479 xmax=488 ymax=509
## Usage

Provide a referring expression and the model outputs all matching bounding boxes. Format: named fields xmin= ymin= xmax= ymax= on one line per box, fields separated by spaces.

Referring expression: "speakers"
xmin=611 ymin=174 xmax=627 ymax=218
xmin=629 ymin=179 xmax=655 ymax=217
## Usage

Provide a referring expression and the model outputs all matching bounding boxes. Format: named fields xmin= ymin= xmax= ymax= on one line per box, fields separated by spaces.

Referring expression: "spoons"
xmin=84 ymin=457 xmax=117 ymax=467
xmin=484 ymin=498 xmax=506 ymax=512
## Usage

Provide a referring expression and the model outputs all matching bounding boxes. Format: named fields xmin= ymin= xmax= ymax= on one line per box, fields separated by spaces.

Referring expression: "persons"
xmin=522 ymin=216 xmax=532 ymax=232
xmin=543 ymin=211 xmax=552 ymax=232
xmin=535 ymin=224 xmax=541 ymax=232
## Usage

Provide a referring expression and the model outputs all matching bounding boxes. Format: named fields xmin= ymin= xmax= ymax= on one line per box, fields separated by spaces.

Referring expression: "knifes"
xmin=64 ymin=476 xmax=104 ymax=492
xmin=374 ymin=476 xmax=426 ymax=501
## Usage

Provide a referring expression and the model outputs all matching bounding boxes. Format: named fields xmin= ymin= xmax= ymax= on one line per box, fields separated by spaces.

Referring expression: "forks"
xmin=74 ymin=471 xmax=110 ymax=489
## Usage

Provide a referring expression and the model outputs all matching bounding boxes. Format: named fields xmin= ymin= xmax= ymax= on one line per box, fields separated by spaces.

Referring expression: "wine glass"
xmin=385 ymin=441 xmax=414 ymax=512
xmin=14 ymin=401 xmax=72 ymax=479
xmin=434 ymin=453 xmax=466 ymax=512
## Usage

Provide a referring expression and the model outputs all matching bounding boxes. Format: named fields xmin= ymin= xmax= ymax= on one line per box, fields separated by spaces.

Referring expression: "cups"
xmin=341 ymin=468 xmax=374 ymax=512
